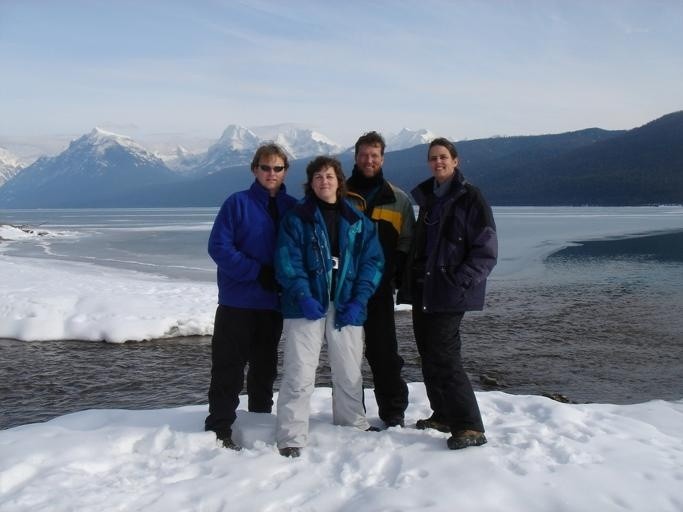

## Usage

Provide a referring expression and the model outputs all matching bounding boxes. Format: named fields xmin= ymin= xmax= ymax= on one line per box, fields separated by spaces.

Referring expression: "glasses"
xmin=261 ymin=165 xmax=284 ymax=172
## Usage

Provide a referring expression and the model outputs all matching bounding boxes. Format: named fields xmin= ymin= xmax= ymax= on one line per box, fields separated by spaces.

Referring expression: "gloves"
xmin=336 ymin=300 xmax=363 ymax=322
xmin=258 ymin=265 xmax=280 ymax=290
xmin=298 ymin=297 xmax=324 ymax=320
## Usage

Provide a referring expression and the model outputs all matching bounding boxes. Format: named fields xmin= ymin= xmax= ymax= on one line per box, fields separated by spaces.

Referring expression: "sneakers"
xmin=447 ymin=429 xmax=487 ymax=449
xmin=221 ymin=438 xmax=240 ymax=451
xmin=366 ymin=426 xmax=380 ymax=431
xmin=279 ymin=447 xmax=299 ymax=457
xmin=383 ymin=412 xmax=404 ymax=427
xmin=416 ymin=419 xmax=451 ymax=433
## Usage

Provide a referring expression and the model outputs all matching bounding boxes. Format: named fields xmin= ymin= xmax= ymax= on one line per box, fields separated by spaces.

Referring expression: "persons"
xmin=343 ymin=130 xmax=418 ymax=429
xmin=275 ymin=156 xmax=385 ymax=458
xmin=205 ymin=141 xmax=299 ymax=451
xmin=395 ymin=138 xmax=498 ymax=449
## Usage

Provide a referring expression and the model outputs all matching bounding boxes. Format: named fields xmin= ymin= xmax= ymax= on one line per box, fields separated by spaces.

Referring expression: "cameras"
xmin=331 ymin=256 xmax=340 ymax=270
